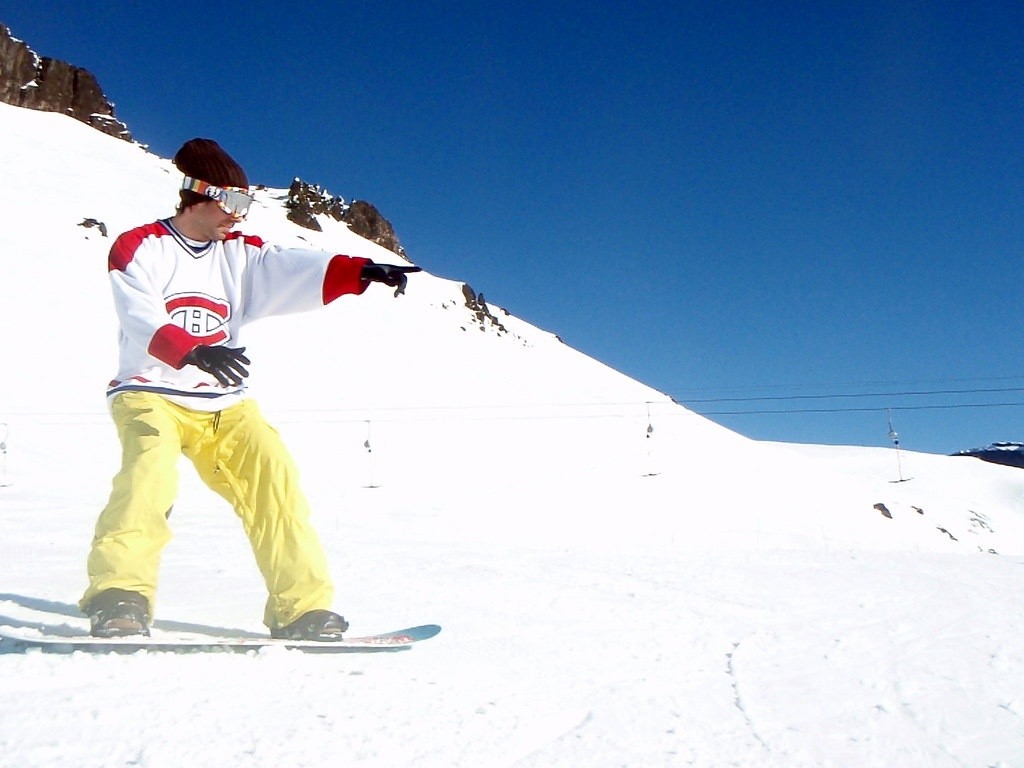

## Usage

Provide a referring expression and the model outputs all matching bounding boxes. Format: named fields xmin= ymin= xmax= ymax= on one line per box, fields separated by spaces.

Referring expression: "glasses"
xmin=182 ymin=177 xmax=251 ymax=218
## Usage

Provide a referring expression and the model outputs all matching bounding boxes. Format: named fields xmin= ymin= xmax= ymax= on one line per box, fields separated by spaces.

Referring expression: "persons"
xmin=79 ymin=137 xmax=423 ymax=640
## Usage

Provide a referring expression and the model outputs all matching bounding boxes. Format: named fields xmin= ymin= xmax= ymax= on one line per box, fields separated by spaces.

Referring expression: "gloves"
xmin=361 ymin=264 xmax=422 ymax=297
xmin=185 ymin=344 xmax=250 ymax=386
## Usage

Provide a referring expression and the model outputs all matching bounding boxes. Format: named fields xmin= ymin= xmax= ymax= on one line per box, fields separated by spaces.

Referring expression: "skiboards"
xmin=0 ymin=614 xmax=443 ymax=654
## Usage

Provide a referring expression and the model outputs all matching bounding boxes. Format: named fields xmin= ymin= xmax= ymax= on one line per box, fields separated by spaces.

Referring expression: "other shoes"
xmin=90 ymin=588 xmax=150 ymax=636
xmin=265 ymin=609 xmax=349 ymax=642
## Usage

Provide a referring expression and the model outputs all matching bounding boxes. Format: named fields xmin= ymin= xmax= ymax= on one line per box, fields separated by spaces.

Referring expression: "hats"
xmin=175 ymin=138 xmax=249 ymax=207
